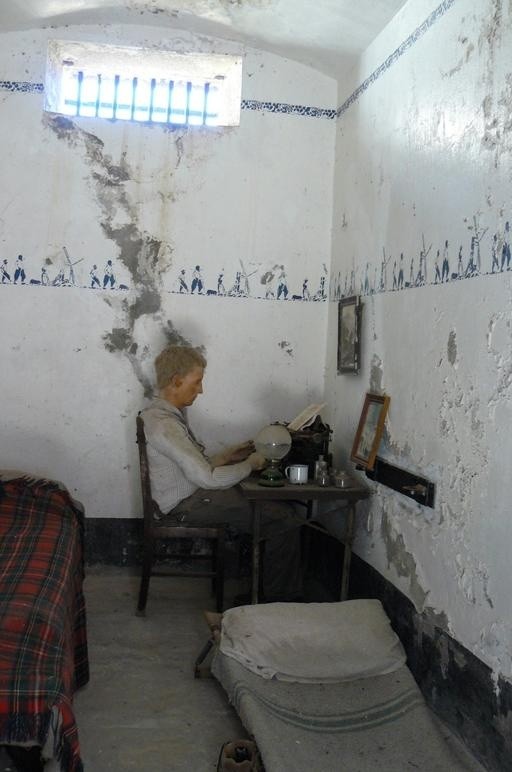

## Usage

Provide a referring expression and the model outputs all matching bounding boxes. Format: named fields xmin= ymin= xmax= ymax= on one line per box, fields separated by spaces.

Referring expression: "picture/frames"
xmin=349 ymin=391 xmax=391 ymax=473
xmin=335 ymin=292 xmax=362 ymax=376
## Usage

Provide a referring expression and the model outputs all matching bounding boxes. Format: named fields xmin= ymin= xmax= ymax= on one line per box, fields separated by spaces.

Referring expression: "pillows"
xmin=216 ymin=596 xmax=409 ymax=686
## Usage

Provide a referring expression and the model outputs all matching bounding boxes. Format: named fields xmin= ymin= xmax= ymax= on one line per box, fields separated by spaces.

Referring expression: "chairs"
xmin=133 ymin=408 xmax=229 ymax=618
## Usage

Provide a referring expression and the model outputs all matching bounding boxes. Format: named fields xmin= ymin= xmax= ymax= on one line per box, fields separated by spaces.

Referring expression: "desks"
xmin=234 ymin=479 xmax=370 ymax=606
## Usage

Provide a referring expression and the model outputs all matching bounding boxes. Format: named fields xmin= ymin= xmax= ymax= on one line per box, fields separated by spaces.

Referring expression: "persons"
xmin=141 ymin=344 xmax=322 ymax=611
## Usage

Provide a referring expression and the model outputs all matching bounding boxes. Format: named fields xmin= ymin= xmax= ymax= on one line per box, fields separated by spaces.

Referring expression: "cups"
xmin=285 ymin=464 xmax=310 ymax=486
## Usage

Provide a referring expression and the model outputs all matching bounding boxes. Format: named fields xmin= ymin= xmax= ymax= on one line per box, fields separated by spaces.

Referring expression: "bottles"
xmin=316 ymin=455 xmax=352 ymax=488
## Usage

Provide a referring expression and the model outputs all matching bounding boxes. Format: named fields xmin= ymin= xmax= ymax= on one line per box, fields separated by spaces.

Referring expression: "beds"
xmin=193 ymin=606 xmax=488 ymax=772
xmin=0 ymin=464 xmax=90 ymax=772
